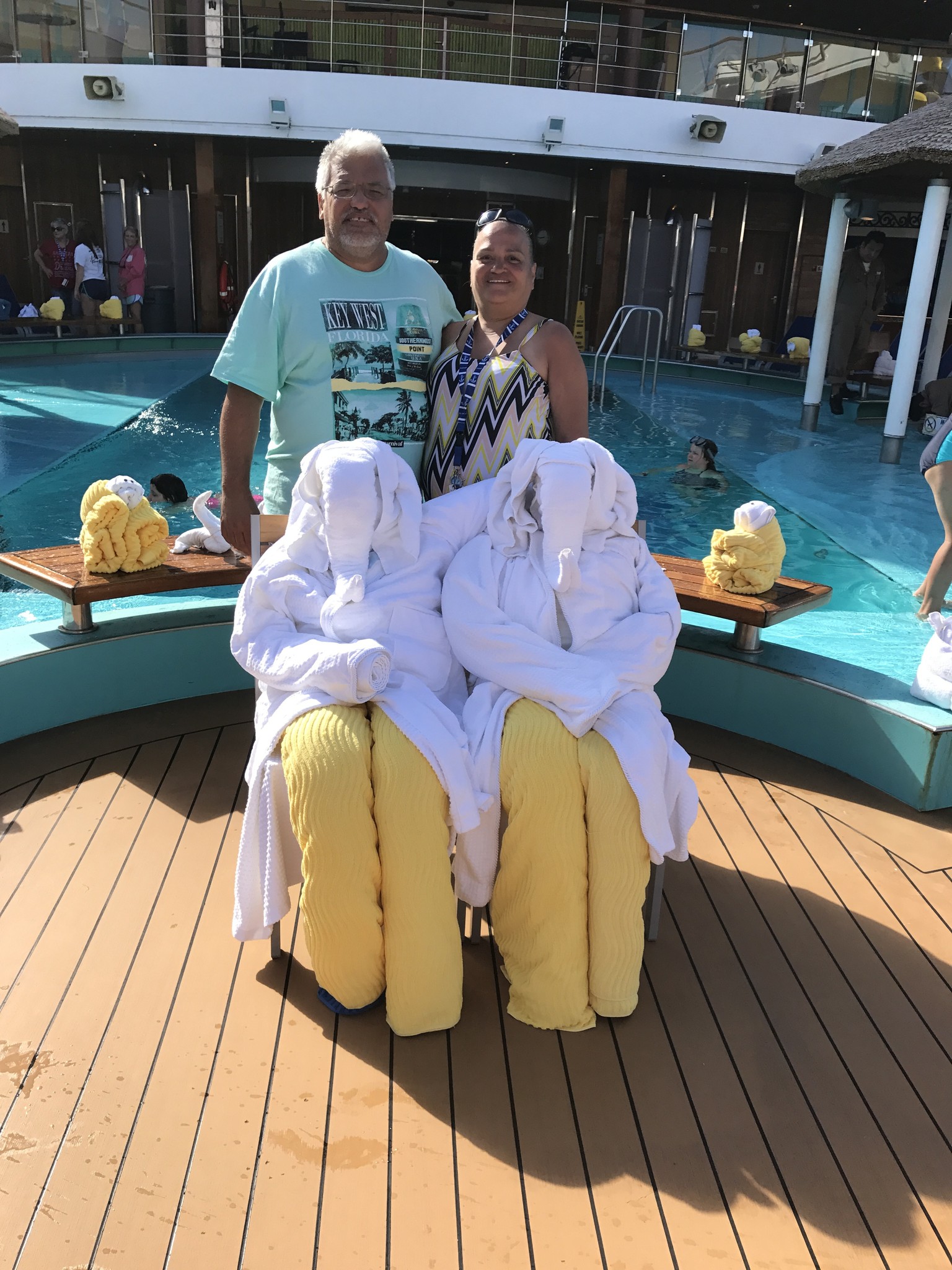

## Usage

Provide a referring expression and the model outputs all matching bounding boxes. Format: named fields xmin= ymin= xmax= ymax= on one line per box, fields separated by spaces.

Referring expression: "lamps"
xmin=267 ymin=94 xmax=291 ymax=129
xmin=775 ymin=58 xmax=798 ymax=77
xmin=542 ymin=115 xmax=567 ymax=148
xmin=748 ymin=61 xmax=769 ymax=83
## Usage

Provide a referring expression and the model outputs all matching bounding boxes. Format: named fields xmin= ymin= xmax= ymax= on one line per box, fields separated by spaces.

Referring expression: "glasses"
xmin=119 ymin=255 xmax=128 ymax=268
xmin=51 ymin=226 xmax=65 ymax=232
xmin=53 ymin=219 xmax=66 ymax=225
xmin=474 ymin=208 xmax=535 ymax=255
xmin=321 ymin=180 xmax=393 ymax=199
xmin=690 ymin=436 xmax=707 ymax=447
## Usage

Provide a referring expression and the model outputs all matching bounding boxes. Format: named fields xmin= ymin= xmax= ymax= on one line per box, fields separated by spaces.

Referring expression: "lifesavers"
xmin=219 ymin=260 xmax=234 ymax=303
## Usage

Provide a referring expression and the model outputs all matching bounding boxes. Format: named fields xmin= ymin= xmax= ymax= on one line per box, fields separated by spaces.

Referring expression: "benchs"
xmin=0 ymin=315 xmax=144 ymax=341
xmin=0 ymin=533 xmax=835 ymax=657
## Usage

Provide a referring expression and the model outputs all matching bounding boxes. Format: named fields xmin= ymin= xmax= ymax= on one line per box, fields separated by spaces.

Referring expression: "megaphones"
xmin=83 ymin=76 xmax=120 ymax=101
xmin=693 ymin=114 xmax=726 ymax=143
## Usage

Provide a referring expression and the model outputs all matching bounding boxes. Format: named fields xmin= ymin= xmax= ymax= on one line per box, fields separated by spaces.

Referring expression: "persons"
xmin=908 ymin=377 xmax=952 ymax=615
xmin=146 ymin=474 xmax=188 ymax=502
xmin=687 ymin=435 xmax=718 ymax=470
xmin=33 ymin=218 xmax=145 ymax=335
xmin=826 ymin=231 xmax=890 ymax=414
xmin=214 ymin=127 xmax=587 ymax=570
xmin=98 ymin=0 xmax=124 ymax=64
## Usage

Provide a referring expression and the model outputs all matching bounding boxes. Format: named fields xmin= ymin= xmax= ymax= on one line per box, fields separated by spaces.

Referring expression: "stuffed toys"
xmin=106 ymin=475 xmax=233 ymax=554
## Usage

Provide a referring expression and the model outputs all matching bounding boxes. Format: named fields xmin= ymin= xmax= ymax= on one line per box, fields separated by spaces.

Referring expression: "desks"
xmin=14 ymin=13 xmax=76 ymax=63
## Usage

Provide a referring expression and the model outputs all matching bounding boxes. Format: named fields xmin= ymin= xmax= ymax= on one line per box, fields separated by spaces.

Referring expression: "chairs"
xmin=243 ymin=498 xmax=672 ymax=958
xmin=846 ymin=328 xmax=931 ymax=393
xmin=763 ymin=311 xmax=814 ymax=374
xmin=0 ymin=273 xmax=72 ymax=337
